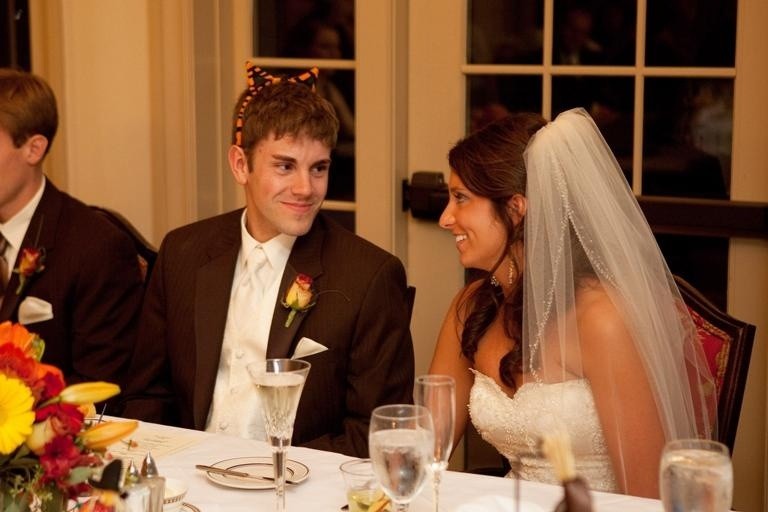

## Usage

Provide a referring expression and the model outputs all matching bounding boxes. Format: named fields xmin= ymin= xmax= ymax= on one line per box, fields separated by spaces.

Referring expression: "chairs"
xmin=668 ymin=273 xmax=756 ymax=458
xmin=89 ymin=205 xmax=159 ymax=288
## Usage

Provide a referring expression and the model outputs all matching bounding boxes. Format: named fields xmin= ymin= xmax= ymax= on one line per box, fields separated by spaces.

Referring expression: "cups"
xmin=659 ymin=437 xmax=732 ymax=512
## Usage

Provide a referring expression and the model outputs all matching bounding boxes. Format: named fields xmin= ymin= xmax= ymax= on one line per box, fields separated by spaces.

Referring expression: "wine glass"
xmin=247 ymin=359 xmax=456 ymax=512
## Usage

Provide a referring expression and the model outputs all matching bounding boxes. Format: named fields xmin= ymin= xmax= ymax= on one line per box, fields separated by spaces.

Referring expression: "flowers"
xmin=0 ymin=320 xmax=139 ymax=512
xmin=11 ymin=249 xmax=45 ymax=295
xmin=279 ymin=272 xmax=317 ymax=328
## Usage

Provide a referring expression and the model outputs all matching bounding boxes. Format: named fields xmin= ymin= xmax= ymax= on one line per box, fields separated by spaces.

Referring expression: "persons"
xmin=404 ymin=106 xmax=721 ymax=509
xmin=123 ymin=61 xmax=416 ymax=470
xmin=0 ymin=68 xmax=144 ymax=415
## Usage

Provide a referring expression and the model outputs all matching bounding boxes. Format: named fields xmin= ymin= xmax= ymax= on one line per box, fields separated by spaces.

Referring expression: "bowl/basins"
xmin=162 ymin=477 xmax=188 ymax=511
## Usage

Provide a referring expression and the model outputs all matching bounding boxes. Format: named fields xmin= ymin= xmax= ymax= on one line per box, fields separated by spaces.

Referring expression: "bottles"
xmin=125 ymin=455 xmax=165 ymax=511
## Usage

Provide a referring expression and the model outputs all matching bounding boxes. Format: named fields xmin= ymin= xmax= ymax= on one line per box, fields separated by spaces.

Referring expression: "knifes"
xmin=194 ymin=464 xmax=299 ymax=486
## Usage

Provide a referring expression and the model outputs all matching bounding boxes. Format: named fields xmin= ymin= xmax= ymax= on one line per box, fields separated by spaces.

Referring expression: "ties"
xmin=0 ymin=233 xmax=8 ymax=308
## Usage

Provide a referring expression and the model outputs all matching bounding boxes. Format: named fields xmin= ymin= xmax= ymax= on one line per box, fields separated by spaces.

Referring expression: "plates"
xmin=206 ymin=457 xmax=309 ymax=490
